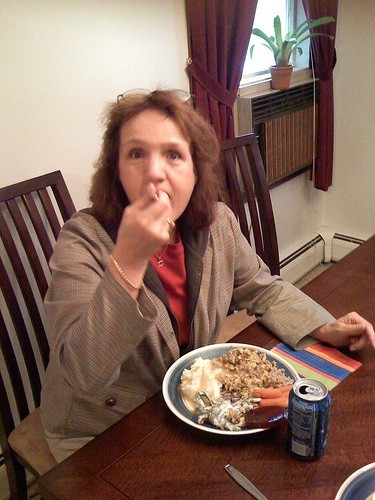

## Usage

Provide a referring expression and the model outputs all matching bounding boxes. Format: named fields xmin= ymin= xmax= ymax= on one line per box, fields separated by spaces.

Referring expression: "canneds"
xmin=288 ymin=379 xmax=331 ymax=460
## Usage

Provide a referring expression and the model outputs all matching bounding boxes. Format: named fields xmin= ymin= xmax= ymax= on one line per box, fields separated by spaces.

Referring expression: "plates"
xmin=334 ymin=463 xmax=375 ymax=499
xmin=161 ymin=342 xmax=300 ymax=435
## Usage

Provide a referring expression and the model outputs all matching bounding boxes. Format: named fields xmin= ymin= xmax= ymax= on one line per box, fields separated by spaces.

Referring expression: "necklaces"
xmin=152 ymin=232 xmax=174 ymax=267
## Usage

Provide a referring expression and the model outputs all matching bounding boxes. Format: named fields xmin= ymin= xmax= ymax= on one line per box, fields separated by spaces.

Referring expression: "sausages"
xmin=253 ymin=384 xmax=293 ymax=407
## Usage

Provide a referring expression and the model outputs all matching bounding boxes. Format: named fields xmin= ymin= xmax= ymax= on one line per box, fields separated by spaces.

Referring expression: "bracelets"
xmin=110 ymin=255 xmax=141 ymax=290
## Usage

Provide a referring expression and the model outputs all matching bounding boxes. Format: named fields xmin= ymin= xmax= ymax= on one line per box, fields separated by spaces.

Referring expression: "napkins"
xmin=270 ymin=341 xmax=363 ymax=393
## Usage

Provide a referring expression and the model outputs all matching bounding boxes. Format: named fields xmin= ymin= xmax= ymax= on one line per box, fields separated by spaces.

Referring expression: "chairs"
xmin=212 ymin=133 xmax=280 ymax=343
xmin=0 ymin=170 xmax=77 ymax=500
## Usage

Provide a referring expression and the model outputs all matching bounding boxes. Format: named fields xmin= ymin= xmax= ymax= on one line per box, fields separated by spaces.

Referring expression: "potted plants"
xmin=249 ymin=14 xmax=335 ymax=89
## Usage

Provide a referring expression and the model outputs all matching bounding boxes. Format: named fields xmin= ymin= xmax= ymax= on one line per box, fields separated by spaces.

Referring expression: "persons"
xmin=39 ymin=88 xmax=375 ymax=464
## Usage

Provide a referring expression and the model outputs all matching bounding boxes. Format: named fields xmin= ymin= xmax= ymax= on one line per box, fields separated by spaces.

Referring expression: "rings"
xmin=167 ymin=217 xmax=173 ymax=224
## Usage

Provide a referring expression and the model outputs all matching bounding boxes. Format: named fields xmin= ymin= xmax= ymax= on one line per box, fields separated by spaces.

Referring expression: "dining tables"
xmin=35 ymin=235 xmax=375 ymax=500
